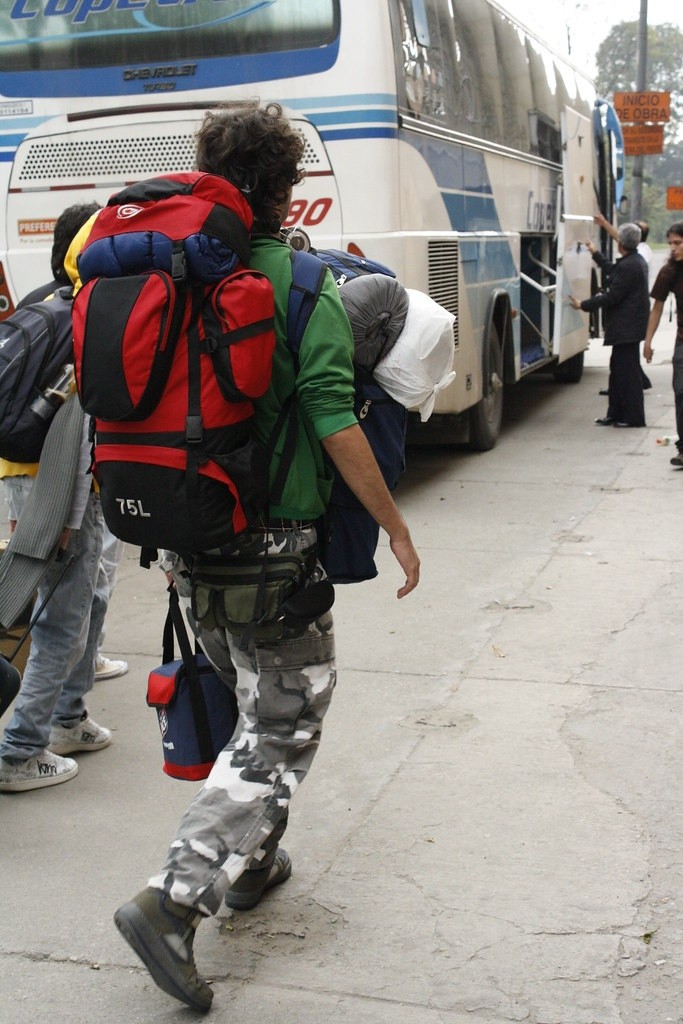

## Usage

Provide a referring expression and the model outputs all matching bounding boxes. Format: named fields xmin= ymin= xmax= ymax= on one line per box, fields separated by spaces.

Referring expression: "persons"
xmin=643 ymin=220 xmax=683 ymax=466
xmin=0 ymin=203 xmax=111 ymax=794
xmin=94 ymin=522 xmax=129 ymax=681
xmin=568 ymin=211 xmax=652 ymax=428
xmin=113 ymin=103 xmax=420 ymax=1014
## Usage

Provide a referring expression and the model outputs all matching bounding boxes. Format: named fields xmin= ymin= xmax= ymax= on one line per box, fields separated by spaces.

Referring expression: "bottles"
xmin=44 ymin=364 xmax=77 ymax=407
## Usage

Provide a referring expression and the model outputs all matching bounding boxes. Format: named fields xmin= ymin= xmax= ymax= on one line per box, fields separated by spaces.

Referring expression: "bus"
xmin=0 ymin=0 xmax=625 ymax=450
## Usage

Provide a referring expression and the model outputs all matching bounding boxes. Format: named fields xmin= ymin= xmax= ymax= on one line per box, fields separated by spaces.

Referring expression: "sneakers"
xmin=93 ymin=657 xmax=130 ymax=681
xmin=0 ymin=747 xmax=79 ymax=791
xmin=46 ymin=716 xmax=114 ymax=755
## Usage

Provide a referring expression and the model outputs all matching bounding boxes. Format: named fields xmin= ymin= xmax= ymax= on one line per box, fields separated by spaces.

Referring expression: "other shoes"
xmin=227 ymin=848 xmax=291 ymax=910
xmin=616 ymin=421 xmax=633 ymax=428
xmin=596 ymin=417 xmax=615 ymax=426
xmin=114 ymin=886 xmax=214 ymax=1013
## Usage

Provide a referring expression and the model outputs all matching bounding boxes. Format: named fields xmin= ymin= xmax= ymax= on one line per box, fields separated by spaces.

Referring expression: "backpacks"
xmin=63 ymin=171 xmax=276 ymax=568
xmin=255 ymin=247 xmax=408 ymax=586
xmin=0 ymin=288 xmax=75 ymax=464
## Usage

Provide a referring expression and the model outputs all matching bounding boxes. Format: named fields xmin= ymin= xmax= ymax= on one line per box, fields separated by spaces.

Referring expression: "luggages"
xmin=1 ymin=546 xmax=77 ymax=718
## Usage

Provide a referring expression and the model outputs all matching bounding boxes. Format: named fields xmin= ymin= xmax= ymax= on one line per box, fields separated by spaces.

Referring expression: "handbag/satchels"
xmin=147 ymin=579 xmax=238 ymax=784
